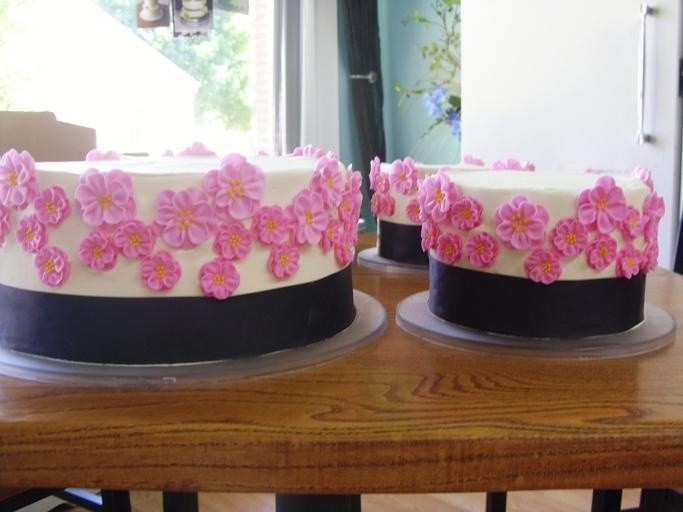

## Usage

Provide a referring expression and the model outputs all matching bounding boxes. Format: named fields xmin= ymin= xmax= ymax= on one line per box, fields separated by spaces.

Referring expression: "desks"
xmin=1 ymin=220 xmax=683 ymax=511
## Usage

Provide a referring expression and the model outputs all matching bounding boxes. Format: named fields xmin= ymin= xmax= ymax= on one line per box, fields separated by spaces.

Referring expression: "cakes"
xmin=417 ymin=166 xmax=665 ymax=338
xmin=180 ymin=1 xmax=209 ymax=21
xmin=143 ymin=0 xmax=160 ymax=17
xmin=369 ymin=155 xmax=534 ymax=265
xmin=0 ymin=143 xmax=363 ymax=363
